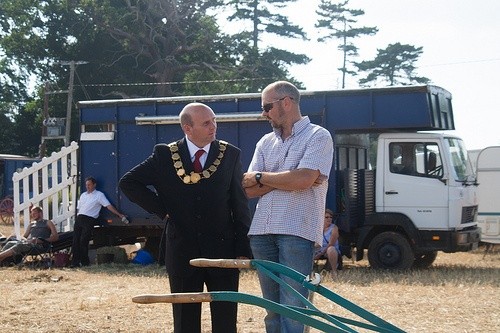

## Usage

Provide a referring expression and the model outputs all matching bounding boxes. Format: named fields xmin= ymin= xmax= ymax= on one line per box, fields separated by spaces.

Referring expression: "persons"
xmin=69 ymin=176 xmax=130 ymax=268
xmin=0 ymin=206 xmax=58 ymax=266
xmin=120 ymin=102 xmax=253 ymax=333
xmin=313 ymin=208 xmax=342 ymax=279
xmin=241 ymin=80 xmax=335 ymax=333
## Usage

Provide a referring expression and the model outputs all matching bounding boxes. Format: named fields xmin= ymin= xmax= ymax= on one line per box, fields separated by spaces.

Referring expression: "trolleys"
xmin=132 ymin=258 xmax=407 ymax=333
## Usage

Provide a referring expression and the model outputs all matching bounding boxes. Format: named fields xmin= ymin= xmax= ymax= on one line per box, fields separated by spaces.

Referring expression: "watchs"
xmin=255 ymin=172 xmax=264 ymax=188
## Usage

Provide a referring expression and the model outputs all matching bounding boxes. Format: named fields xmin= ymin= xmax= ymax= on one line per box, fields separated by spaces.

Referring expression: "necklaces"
xmin=169 ymin=139 xmax=228 ymax=185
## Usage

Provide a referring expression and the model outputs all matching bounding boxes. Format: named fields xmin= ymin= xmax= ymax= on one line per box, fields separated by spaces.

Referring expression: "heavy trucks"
xmin=12 ymin=84 xmax=482 ymax=271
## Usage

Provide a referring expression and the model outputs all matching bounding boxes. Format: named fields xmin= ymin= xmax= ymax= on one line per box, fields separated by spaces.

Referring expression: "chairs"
xmin=19 ymin=221 xmax=58 ymax=267
xmin=428 ymin=152 xmax=439 ymax=175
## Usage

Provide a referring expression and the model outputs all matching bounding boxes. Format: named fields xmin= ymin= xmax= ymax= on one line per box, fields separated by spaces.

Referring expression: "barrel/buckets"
xmin=97 ymin=254 xmax=114 ymax=264
xmin=131 ymin=249 xmax=154 ymax=266
xmin=53 ymin=254 xmax=70 ymax=267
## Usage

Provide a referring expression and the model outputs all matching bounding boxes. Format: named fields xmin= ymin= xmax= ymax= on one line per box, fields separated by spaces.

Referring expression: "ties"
xmin=193 ymin=150 xmax=204 ymax=173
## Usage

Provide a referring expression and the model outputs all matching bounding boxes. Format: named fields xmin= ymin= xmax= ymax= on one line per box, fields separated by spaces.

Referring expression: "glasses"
xmin=262 ymin=96 xmax=293 ymax=112
xmin=325 ymin=217 xmax=333 ymax=218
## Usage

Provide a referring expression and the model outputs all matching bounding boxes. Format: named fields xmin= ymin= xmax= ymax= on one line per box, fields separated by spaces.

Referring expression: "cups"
xmin=122 ymin=217 xmax=129 ymax=224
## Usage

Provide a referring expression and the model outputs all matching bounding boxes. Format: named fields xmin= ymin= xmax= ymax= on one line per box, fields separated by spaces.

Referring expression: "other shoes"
xmin=70 ymin=257 xmax=82 ymax=268
